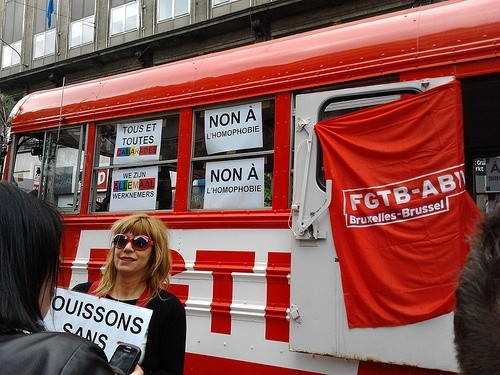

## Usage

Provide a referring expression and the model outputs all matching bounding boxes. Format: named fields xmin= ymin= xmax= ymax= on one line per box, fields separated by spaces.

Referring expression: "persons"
xmin=71 ymin=213 xmax=187 ymax=375
xmin=453 ymin=202 xmax=500 ymax=375
xmin=0 ymin=180 xmax=144 ymax=375
xmin=0 ymin=135 xmax=7 ymax=180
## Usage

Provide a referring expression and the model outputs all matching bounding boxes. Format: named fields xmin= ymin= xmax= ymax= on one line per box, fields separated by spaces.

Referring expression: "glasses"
xmin=113 ymin=233 xmax=155 ymax=250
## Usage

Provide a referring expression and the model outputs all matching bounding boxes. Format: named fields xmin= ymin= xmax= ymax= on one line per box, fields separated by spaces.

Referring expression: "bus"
xmin=0 ymin=0 xmax=500 ymax=375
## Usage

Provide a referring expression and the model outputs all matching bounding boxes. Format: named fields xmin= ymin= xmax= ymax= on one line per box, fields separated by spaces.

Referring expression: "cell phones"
xmin=108 ymin=343 xmax=142 ymax=375
